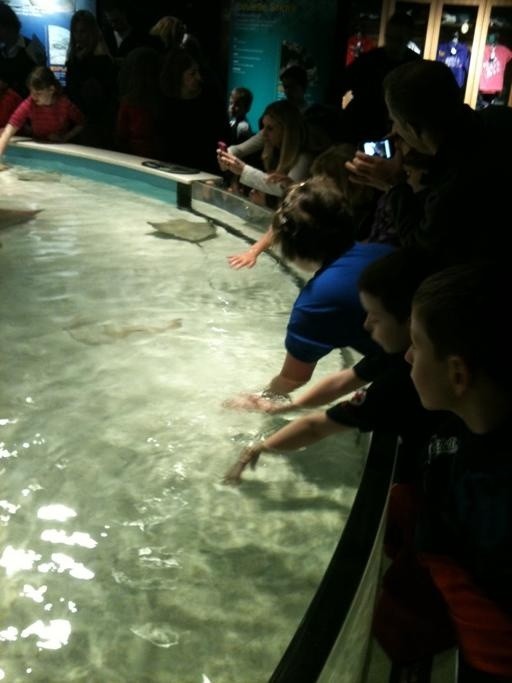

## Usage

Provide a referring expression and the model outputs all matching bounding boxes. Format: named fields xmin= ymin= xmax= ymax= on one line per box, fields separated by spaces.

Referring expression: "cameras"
xmin=362 ymin=137 xmax=392 ymax=163
xmin=218 ymin=141 xmax=226 ymax=152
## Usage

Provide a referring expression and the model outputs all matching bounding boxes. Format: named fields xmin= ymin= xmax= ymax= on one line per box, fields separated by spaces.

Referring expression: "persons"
xmin=216 ymin=35 xmax=512 ymax=673
xmin=1 ymin=4 xmax=213 ymax=158
